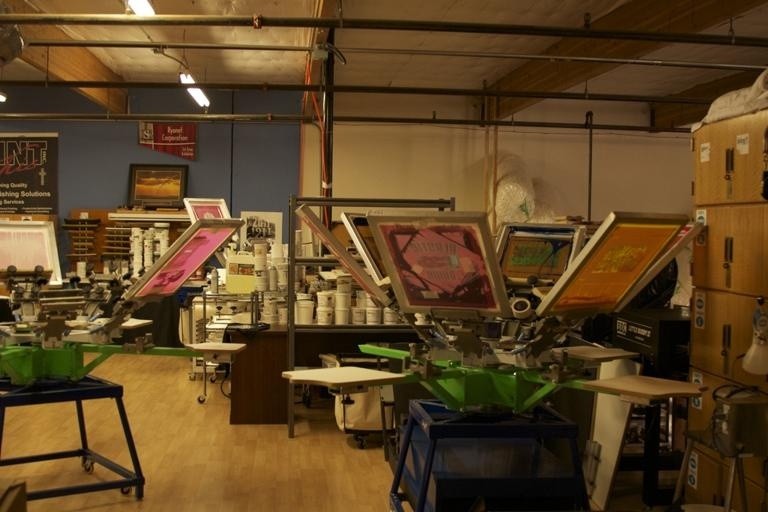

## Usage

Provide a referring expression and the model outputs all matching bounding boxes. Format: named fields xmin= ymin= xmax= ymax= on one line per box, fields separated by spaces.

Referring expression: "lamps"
xmin=176 ymin=64 xmax=210 ymax=111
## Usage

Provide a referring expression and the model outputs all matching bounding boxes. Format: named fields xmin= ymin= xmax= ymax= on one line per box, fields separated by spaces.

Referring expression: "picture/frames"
xmin=124 ymin=163 xmax=285 ymax=304
xmin=297 ymin=204 xmax=704 ymax=324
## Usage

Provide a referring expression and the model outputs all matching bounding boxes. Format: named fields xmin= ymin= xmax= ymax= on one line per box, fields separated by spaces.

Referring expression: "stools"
xmin=282 ymin=365 xmax=413 ymax=442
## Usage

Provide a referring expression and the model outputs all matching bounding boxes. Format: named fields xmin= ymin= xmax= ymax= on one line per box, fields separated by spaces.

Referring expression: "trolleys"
xmin=187 ymin=287 xmax=264 ymax=404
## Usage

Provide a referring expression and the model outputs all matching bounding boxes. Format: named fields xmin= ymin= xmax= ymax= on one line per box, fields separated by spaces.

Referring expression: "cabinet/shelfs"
xmin=227 ymin=322 xmax=286 ymax=424
xmin=690 ymin=70 xmax=768 ymax=512
xmin=388 ymin=400 xmax=590 ymax=512
